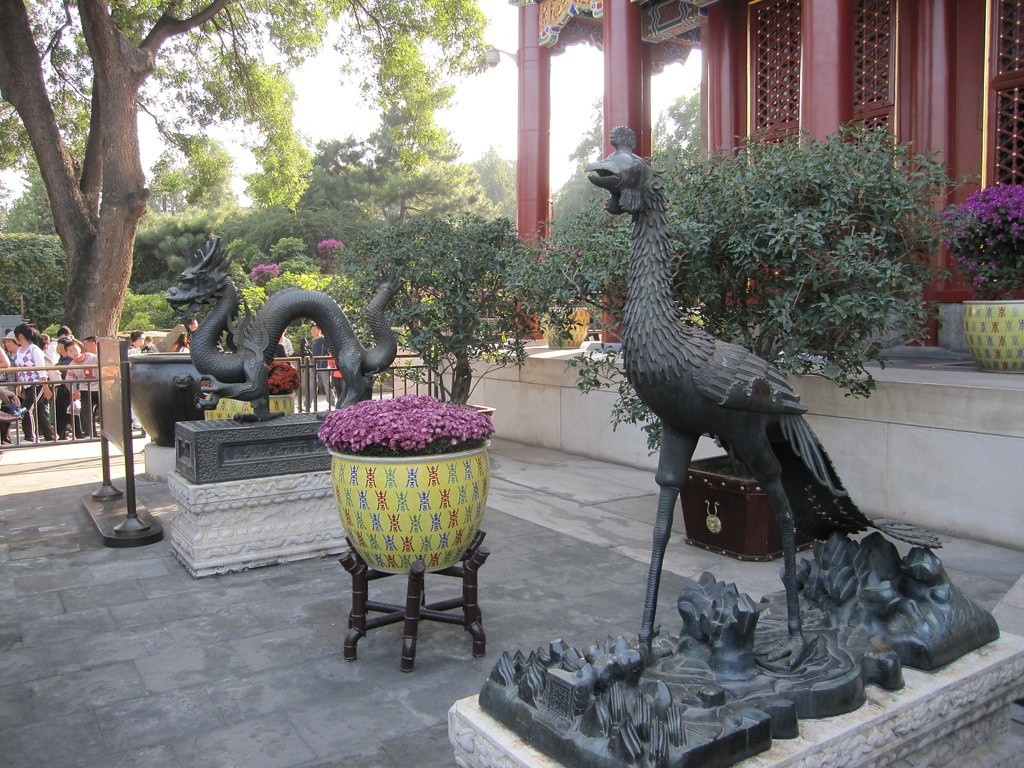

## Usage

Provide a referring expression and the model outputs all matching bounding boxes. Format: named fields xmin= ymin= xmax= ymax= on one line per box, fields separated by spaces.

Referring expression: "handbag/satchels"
xmin=66 ymin=401 xmax=82 ymax=415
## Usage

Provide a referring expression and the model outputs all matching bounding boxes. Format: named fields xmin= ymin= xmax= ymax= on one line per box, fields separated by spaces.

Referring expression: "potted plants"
xmin=344 ymin=115 xmax=933 ymax=564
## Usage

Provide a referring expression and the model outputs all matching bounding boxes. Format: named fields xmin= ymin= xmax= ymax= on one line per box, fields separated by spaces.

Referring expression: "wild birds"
xmin=584 ymin=126 xmax=943 ymax=670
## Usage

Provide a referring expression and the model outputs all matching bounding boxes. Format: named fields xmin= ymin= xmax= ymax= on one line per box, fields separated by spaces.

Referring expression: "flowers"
xmin=947 ymin=189 xmax=1023 ymax=299
xmin=202 ymin=362 xmax=299 ymax=394
xmin=320 ymin=402 xmax=494 ymax=451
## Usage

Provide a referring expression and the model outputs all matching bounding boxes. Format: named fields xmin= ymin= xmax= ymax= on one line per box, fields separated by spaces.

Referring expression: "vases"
xmin=331 ymin=454 xmax=493 ymax=571
xmin=205 ymin=388 xmax=295 ymax=422
xmin=962 ymin=301 xmax=1024 ymax=369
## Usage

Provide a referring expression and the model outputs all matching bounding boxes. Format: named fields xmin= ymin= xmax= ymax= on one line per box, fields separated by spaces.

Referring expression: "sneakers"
xmin=0 ymin=435 xmax=60 ymax=446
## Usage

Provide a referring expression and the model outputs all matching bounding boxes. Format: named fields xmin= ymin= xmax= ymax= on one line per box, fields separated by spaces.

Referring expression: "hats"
xmin=4 ymin=331 xmax=15 ymax=340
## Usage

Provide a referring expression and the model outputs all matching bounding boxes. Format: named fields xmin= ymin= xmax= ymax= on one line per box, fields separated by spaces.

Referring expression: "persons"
xmin=1 ymin=317 xmax=343 ymax=448
